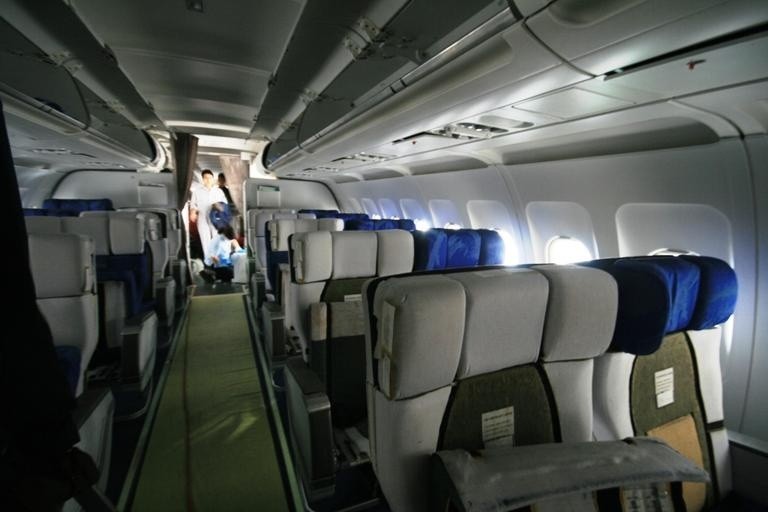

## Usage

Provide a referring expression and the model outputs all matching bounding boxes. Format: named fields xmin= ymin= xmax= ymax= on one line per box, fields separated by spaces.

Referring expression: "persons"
xmin=217 ymin=172 xmax=233 ymax=203
xmin=189 ymin=168 xmax=228 ymax=266
xmin=199 ymin=224 xmax=243 ymax=284
